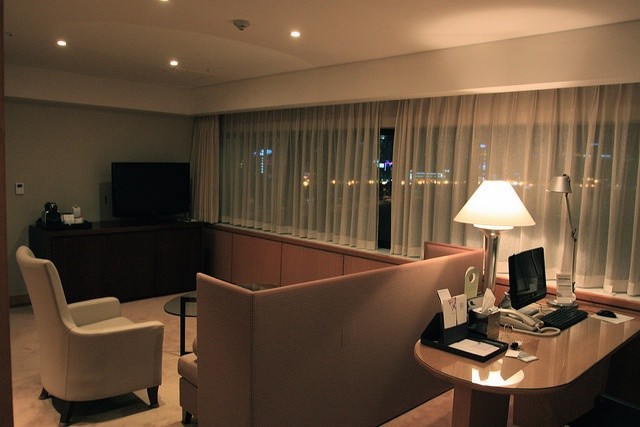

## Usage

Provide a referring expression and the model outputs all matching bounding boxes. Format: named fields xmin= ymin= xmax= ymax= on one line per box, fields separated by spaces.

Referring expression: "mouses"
xmin=598 ymin=307 xmax=617 ymax=320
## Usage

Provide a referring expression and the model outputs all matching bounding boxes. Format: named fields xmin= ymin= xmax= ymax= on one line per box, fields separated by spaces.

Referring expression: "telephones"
xmin=491 ymin=308 xmax=561 ymax=337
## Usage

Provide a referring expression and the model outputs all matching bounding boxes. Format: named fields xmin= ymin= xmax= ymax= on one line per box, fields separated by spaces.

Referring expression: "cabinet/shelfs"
xmin=28 ymin=221 xmax=211 ymax=302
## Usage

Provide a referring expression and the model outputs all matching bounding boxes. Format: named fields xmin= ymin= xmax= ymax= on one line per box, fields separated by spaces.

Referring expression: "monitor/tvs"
xmin=499 ymin=248 xmax=548 ymax=323
xmin=110 ymin=161 xmax=193 ymax=225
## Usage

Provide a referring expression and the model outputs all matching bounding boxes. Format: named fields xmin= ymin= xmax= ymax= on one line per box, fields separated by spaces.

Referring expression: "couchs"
xmin=178 ymin=242 xmax=484 ymax=427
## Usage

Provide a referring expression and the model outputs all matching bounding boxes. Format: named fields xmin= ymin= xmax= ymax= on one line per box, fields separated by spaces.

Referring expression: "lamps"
xmin=453 ymin=179 xmax=535 ymax=296
xmin=547 ymin=174 xmax=579 ymax=307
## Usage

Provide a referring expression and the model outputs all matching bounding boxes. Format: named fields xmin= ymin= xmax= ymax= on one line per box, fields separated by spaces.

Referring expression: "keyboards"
xmin=539 ymin=305 xmax=589 ymax=332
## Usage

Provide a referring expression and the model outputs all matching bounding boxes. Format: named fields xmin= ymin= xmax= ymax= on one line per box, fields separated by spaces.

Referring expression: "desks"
xmin=414 ymin=295 xmax=640 ymax=427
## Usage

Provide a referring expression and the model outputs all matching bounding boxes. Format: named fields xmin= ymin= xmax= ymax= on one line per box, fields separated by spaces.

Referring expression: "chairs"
xmin=16 ymin=245 xmax=164 ymax=427
